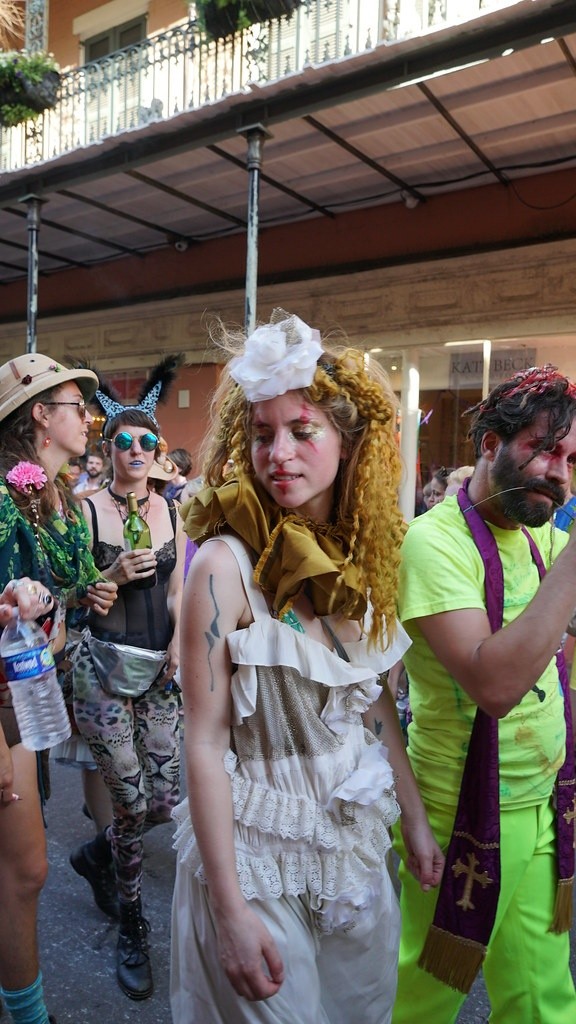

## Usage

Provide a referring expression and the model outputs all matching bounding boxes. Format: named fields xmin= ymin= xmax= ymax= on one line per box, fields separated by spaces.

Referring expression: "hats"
xmin=0 ymin=353 xmax=99 ymax=420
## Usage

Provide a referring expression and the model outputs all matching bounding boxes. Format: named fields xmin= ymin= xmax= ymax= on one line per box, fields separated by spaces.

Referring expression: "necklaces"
xmin=108 ymin=485 xmax=150 ymax=545
xmin=24 ymin=483 xmax=79 ymax=590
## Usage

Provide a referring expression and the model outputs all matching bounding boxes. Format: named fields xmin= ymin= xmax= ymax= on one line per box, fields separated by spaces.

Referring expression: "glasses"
xmin=40 ymin=401 xmax=87 ymax=417
xmin=104 ymin=432 xmax=160 ymax=451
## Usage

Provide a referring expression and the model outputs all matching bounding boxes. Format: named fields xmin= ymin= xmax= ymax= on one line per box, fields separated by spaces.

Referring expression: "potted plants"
xmin=187 ymin=0 xmax=295 ymax=38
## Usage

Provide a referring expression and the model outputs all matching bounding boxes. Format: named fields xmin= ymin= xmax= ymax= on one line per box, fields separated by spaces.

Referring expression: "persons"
xmin=170 ymin=305 xmax=446 ymax=1024
xmin=67 ymin=462 xmax=81 ymax=488
xmin=75 ymin=450 xmax=112 ymax=490
xmin=179 ymin=455 xmax=235 ymax=503
xmin=390 ymin=361 xmax=576 ymax=1024
xmin=0 ymin=352 xmax=118 ymax=1024
xmin=161 ymin=449 xmax=192 ymax=503
xmin=62 ymin=404 xmax=188 ymax=997
xmin=415 ymin=463 xmax=454 ymax=517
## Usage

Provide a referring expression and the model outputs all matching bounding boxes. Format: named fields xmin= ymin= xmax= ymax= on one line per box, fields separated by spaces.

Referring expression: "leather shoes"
xmin=69 ymin=845 xmax=120 ymax=919
xmin=118 ymin=929 xmax=153 ymax=999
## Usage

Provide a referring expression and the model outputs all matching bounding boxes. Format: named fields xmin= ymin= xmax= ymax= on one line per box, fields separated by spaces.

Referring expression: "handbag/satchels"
xmin=87 ymin=637 xmax=167 ymax=697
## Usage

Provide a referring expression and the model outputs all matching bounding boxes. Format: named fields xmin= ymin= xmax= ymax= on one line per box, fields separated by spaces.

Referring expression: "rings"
xmin=42 ymin=591 xmax=51 ymax=607
xmin=25 ymin=583 xmax=37 ymax=594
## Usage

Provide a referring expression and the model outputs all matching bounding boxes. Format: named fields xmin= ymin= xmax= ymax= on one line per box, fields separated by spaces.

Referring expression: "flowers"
xmin=0 ymin=48 xmax=53 ymax=127
xmin=6 ymin=461 xmax=45 ymax=494
xmin=232 ymin=314 xmax=322 ymax=401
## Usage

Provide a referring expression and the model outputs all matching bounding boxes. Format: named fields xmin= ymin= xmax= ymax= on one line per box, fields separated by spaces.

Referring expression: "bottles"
xmin=122 ymin=491 xmax=157 ymax=589
xmin=1 ymin=606 xmax=72 ymax=752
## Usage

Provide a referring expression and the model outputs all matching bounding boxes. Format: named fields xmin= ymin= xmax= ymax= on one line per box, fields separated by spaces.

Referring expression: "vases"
xmin=6 ymin=69 xmax=59 ymax=111
